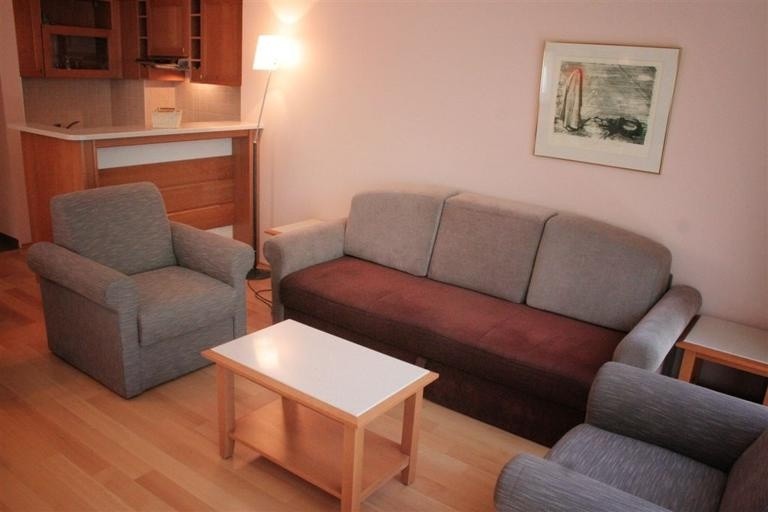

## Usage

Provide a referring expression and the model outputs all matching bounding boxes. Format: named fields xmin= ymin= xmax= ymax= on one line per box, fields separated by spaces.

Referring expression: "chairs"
xmin=25 ymin=181 xmax=256 ymax=400
xmin=491 ymin=361 xmax=768 ymax=512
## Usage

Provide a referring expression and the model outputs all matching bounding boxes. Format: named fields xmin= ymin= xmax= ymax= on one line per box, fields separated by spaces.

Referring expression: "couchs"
xmin=265 ymin=180 xmax=703 ymax=448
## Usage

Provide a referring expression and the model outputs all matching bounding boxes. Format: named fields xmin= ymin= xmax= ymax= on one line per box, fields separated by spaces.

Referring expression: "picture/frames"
xmin=533 ymin=39 xmax=682 ymax=177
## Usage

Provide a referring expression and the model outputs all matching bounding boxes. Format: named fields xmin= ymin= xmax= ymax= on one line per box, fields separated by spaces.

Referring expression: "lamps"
xmin=244 ymin=34 xmax=303 ymax=149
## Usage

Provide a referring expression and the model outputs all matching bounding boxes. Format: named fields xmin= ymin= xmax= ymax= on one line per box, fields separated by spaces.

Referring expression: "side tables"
xmin=677 ymin=312 xmax=768 ymax=408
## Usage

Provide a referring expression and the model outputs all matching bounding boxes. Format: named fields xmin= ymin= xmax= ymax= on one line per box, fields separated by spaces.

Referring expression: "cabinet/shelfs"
xmin=12 ymin=1 xmax=265 ymax=270
